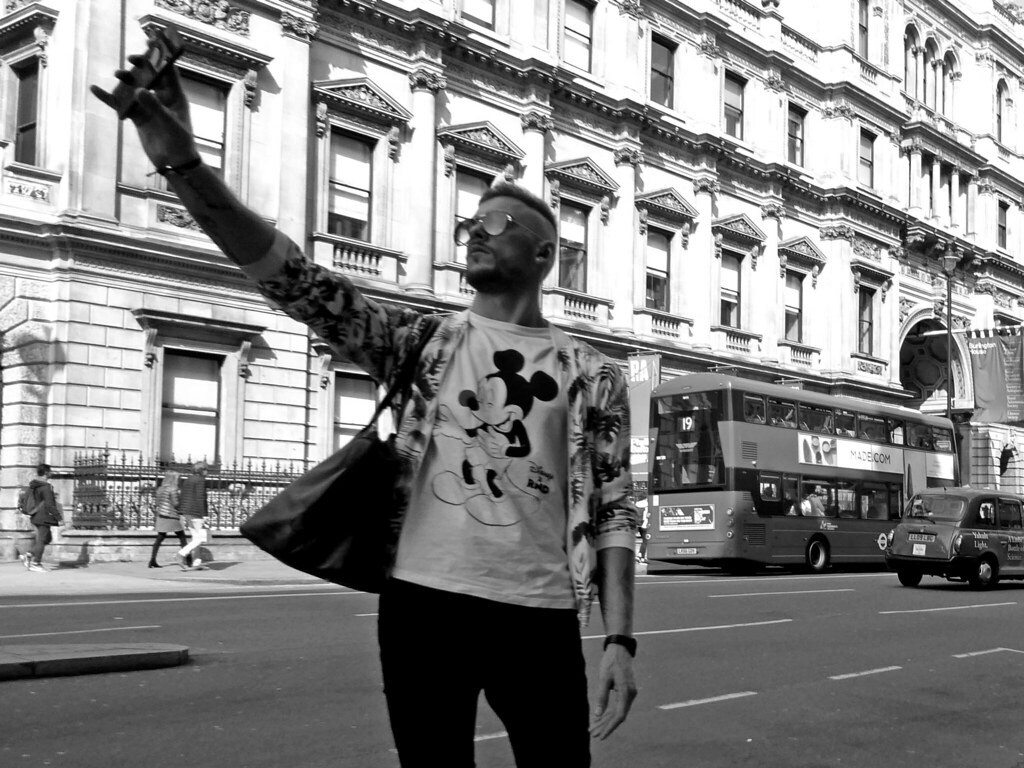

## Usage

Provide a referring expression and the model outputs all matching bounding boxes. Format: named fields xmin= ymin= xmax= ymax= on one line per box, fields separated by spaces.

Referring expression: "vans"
xmin=884 ymin=487 xmax=1024 ymax=593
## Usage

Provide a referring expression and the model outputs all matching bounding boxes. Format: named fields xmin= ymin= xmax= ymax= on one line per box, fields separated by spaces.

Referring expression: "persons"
xmin=802 ymin=491 xmax=827 ymax=516
xmin=89 ymin=13 xmax=640 ymax=768
xmin=174 ymin=460 xmax=210 ymax=572
xmin=761 ymin=486 xmax=774 ymax=501
xmin=18 ymin=464 xmax=62 ymax=573
xmin=147 ymin=468 xmax=201 ymax=569
xmin=866 ymin=427 xmax=876 ymax=440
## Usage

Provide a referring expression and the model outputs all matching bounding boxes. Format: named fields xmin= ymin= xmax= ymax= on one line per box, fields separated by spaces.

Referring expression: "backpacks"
xmin=18 ymin=484 xmax=45 ymax=516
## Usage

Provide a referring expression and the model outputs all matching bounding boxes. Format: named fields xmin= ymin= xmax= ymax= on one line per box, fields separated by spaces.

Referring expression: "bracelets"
xmin=154 ymin=157 xmax=204 ymax=178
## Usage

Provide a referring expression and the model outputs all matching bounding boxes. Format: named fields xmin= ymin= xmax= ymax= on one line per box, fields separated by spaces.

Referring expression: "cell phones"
xmin=113 ymin=26 xmax=187 ymax=119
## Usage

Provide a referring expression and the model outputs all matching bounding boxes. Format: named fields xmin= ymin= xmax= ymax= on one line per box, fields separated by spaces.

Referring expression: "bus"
xmin=646 ymin=371 xmax=959 ymax=575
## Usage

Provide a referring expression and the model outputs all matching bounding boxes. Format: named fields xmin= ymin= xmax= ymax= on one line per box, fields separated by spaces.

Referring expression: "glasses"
xmin=453 ymin=209 xmax=544 ymax=246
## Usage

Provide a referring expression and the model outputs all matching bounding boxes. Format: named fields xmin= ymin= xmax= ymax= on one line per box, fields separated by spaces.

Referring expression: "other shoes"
xmin=193 ymin=565 xmax=210 ymax=570
xmin=148 ymin=558 xmax=162 ymax=568
xmin=175 ymin=553 xmax=189 ymax=569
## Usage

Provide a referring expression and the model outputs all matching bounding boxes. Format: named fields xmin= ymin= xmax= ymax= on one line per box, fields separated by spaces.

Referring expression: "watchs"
xmin=601 ymin=633 xmax=637 ymax=658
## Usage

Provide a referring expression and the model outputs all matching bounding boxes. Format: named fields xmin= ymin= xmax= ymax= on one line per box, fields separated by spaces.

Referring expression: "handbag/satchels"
xmin=239 ymin=316 xmax=442 ymax=595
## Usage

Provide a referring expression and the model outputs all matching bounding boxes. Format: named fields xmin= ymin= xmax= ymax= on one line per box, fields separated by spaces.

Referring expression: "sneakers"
xmin=29 ymin=564 xmax=51 ymax=573
xmin=18 ymin=552 xmax=30 ymax=568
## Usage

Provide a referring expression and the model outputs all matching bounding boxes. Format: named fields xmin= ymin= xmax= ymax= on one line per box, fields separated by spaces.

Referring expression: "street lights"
xmin=937 ymin=241 xmax=961 ymax=421
